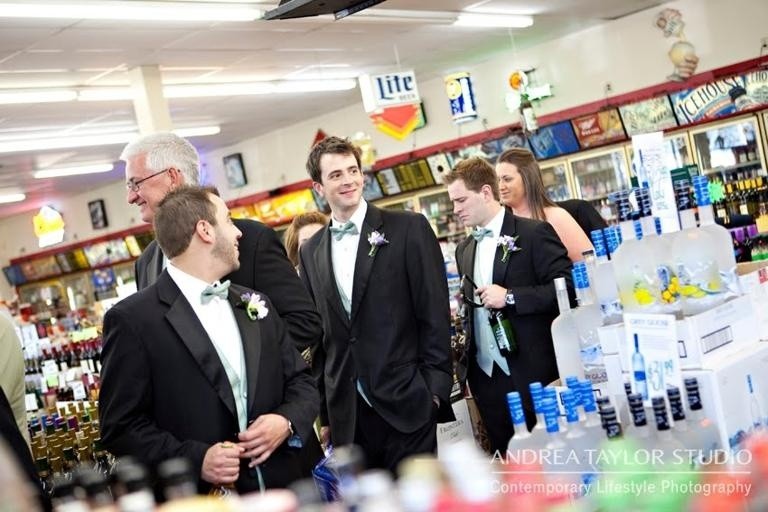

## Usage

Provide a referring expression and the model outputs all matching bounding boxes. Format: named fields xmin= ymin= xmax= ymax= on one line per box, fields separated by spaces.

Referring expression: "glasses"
xmin=127 ymin=169 xmax=169 ymax=191
xmin=460 ymin=274 xmax=485 ymax=308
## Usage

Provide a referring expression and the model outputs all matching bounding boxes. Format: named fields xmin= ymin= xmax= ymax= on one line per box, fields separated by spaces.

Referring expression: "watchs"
xmin=288 ymin=419 xmax=297 ymax=437
xmin=506 ymin=289 xmax=516 ymax=306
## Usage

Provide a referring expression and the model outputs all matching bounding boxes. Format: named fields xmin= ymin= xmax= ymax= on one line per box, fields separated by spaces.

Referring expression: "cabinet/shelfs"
xmin=8 ymin=107 xmax=768 ymax=338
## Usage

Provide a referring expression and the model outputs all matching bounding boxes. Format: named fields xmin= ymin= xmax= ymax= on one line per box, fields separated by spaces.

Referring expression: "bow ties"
xmin=201 ymin=280 xmax=231 ymax=305
xmin=328 ymin=221 xmax=358 ymax=241
xmin=472 ymin=228 xmax=494 ymax=241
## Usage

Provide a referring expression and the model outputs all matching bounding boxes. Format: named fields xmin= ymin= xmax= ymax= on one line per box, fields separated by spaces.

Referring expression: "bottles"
xmin=550 ymin=176 xmax=739 ymax=388
xmin=19 ymin=323 xmax=107 ymax=491
xmin=632 ymin=333 xmax=648 ymax=399
xmin=383 ymin=194 xmax=467 ymax=236
xmin=50 ymin=421 xmax=768 ymax=511
xmin=708 ymin=168 xmax=768 ymax=263
xmin=505 ymin=368 xmax=722 ymax=488
xmin=488 ymin=307 xmax=527 ymax=359
xmin=450 ymin=313 xmax=465 ymax=384
xmin=638 ymin=149 xmax=653 ymax=206
xmin=745 ymin=374 xmax=764 ymax=430
xmin=519 ymin=93 xmax=540 ymax=138
xmin=727 ymin=88 xmax=762 ymax=112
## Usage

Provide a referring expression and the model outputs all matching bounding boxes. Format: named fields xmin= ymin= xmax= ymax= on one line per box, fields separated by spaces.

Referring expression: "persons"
xmin=98 ymin=182 xmax=324 ymax=506
xmin=298 ymin=136 xmax=456 ymax=480
xmin=119 ymin=132 xmax=326 ymax=485
xmin=441 ymin=156 xmax=578 ymax=461
xmin=495 ymin=149 xmax=594 ymax=262
xmin=554 ymin=199 xmax=608 ymax=247
xmin=280 ymin=212 xmax=329 ymax=278
xmin=0 ymin=312 xmax=57 ymax=512
